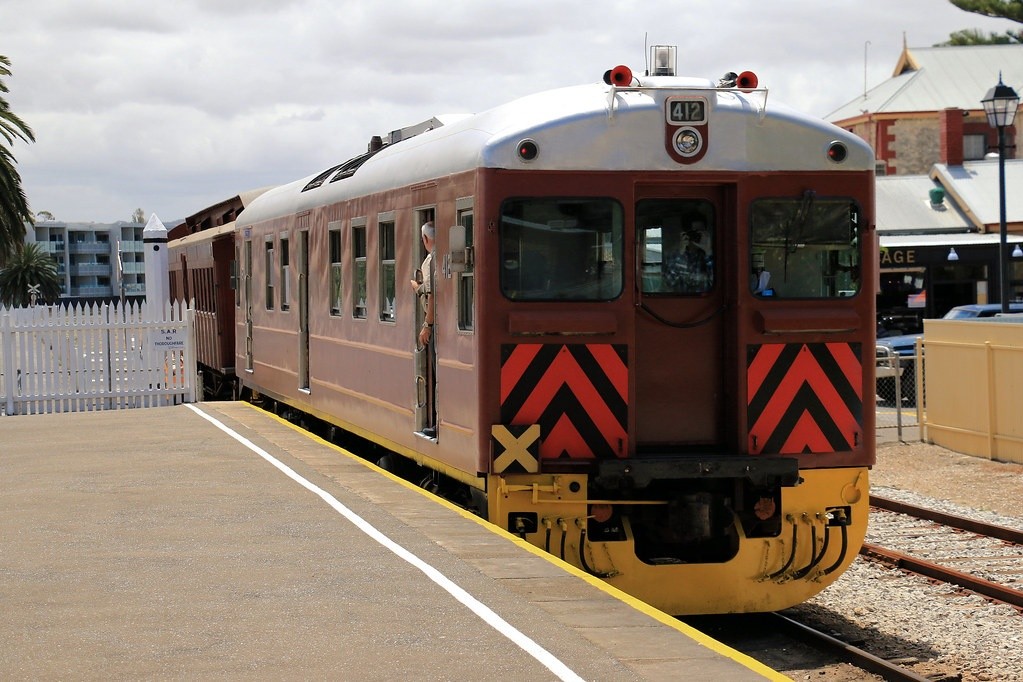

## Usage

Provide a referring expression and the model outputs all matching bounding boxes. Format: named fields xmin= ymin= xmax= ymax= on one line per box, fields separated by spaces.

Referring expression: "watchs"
xmin=422 ymin=322 xmax=433 ymax=328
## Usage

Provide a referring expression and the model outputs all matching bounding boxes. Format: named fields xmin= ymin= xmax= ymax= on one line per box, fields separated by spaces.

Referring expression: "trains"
xmin=167 ymin=33 xmax=876 ymax=616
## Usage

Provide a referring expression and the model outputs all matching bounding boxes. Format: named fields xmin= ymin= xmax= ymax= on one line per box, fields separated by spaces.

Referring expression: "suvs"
xmin=876 ymin=303 xmax=1023 ymax=407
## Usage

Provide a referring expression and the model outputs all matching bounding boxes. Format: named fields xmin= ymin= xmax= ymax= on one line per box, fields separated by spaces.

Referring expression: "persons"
xmin=663 ymin=210 xmax=714 ymax=296
xmin=410 ymin=220 xmax=437 ymax=439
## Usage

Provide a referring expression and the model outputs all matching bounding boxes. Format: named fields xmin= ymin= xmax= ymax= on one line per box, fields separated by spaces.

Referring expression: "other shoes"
xmin=423 ymin=426 xmax=436 ymax=437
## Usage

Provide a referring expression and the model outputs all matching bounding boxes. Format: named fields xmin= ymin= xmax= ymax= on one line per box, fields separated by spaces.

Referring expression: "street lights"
xmin=980 ymin=68 xmax=1021 ymax=313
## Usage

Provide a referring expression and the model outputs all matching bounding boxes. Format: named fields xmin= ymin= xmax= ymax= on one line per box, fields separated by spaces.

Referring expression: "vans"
xmin=875 ymin=315 xmax=923 ymax=336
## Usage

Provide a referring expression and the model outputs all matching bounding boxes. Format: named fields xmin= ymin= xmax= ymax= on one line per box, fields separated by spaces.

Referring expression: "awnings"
xmin=879 ymin=232 xmax=1023 ymax=247
